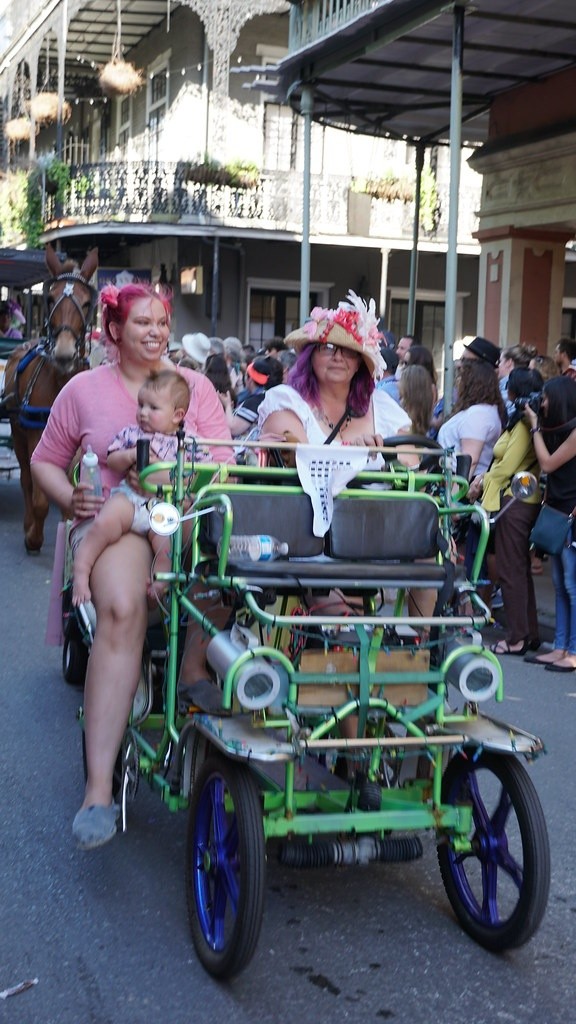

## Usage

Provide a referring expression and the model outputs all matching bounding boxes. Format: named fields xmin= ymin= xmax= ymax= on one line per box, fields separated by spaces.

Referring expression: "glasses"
xmin=316 ymin=343 xmax=360 ymax=358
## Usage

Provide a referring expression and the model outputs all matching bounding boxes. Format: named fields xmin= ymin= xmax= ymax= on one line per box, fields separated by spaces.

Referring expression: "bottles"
xmin=217 ymin=533 xmax=289 ymax=562
xmin=81 ymin=445 xmax=103 ymax=498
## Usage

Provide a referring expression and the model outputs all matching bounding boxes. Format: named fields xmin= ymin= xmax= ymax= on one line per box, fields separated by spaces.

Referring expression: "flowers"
xmin=302 ymin=292 xmax=392 ymax=382
xmin=99 ymin=284 xmax=119 ymax=308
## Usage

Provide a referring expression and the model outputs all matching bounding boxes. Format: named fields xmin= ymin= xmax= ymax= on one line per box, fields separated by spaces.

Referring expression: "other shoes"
xmin=545 ymin=663 xmax=576 ymax=671
xmin=176 ymin=679 xmax=230 ymax=716
xmin=72 ymin=797 xmax=119 ymax=850
xmin=524 ymin=654 xmax=552 ymax=665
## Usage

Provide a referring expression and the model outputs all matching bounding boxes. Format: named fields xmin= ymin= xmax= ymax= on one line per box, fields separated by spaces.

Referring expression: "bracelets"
xmin=530 ymin=426 xmax=541 ymax=432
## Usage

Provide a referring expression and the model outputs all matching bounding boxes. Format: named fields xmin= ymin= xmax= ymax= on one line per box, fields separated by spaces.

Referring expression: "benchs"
xmin=201 ymin=492 xmax=469 ymax=584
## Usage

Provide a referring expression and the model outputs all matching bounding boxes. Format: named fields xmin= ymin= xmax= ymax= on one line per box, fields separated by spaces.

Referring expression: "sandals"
xmin=489 ymin=640 xmax=528 ymax=655
xmin=528 ymin=638 xmax=541 ymax=651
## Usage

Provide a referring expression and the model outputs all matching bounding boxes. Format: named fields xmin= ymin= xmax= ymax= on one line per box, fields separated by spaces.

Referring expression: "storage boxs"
xmin=297 ymin=645 xmax=431 ymax=709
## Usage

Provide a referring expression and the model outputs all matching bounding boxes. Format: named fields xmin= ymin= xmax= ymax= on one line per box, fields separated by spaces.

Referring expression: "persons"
xmin=163 ymin=332 xmax=298 ymax=463
xmin=0 ymin=301 xmax=23 ymax=340
xmin=374 ymin=315 xmax=576 ymax=672
xmin=30 ymin=284 xmax=239 ymax=851
xmin=70 ymin=360 xmax=212 ymax=607
xmin=254 ymin=287 xmax=458 ymax=739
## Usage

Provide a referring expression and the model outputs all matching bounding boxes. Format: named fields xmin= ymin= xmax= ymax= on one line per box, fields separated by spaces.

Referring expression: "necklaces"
xmin=324 ymin=414 xmax=351 ymax=441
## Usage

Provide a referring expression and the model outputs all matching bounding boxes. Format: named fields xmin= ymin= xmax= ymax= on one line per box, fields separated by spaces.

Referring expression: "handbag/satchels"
xmin=528 ymin=502 xmax=571 ymax=555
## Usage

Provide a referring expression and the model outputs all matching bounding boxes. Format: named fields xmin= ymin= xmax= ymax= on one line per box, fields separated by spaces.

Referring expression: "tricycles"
xmin=62 ymin=417 xmax=552 ymax=982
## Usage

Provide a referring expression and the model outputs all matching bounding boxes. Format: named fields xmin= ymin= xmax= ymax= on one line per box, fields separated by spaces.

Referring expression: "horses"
xmin=3 ymin=244 xmax=99 ymax=557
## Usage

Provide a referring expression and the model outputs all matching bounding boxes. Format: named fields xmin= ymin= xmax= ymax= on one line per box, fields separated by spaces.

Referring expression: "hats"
xmin=463 ymin=337 xmax=501 ymax=368
xmin=182 ymin=332 xmax=212 ymax=363
xmin=282 ymin=289 xmax=388 ymax=384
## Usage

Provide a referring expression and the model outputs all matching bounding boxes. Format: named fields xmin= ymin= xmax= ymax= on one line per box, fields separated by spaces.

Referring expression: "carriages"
xmin=0 ymin=243 xmax=98 ymax=558
